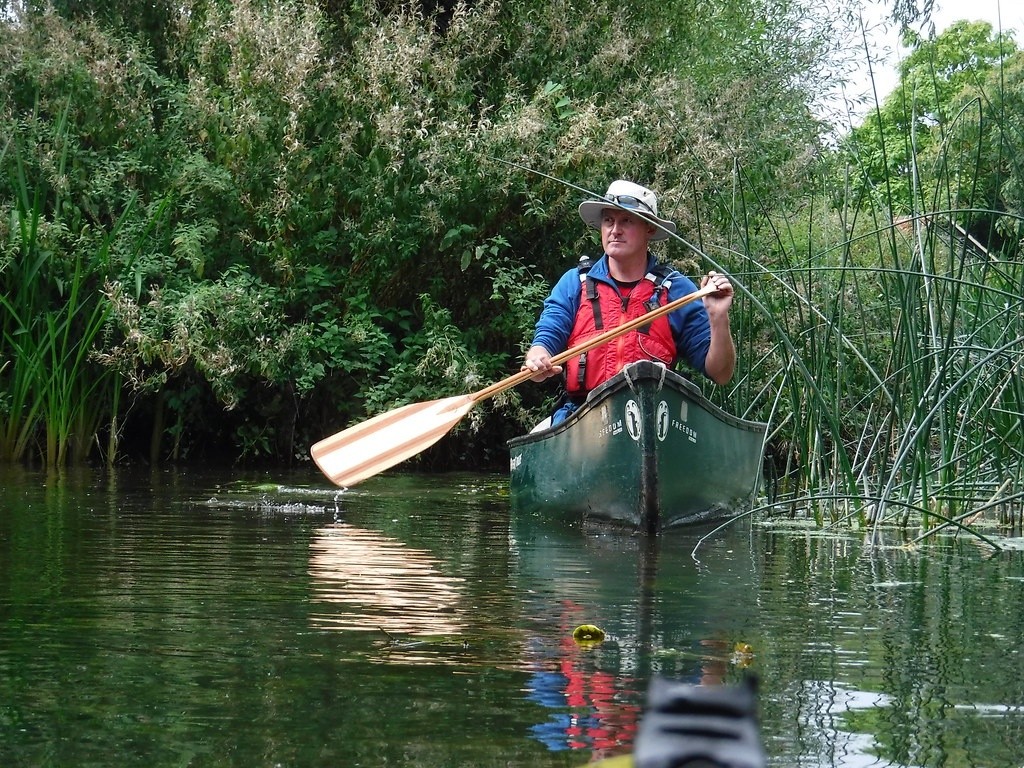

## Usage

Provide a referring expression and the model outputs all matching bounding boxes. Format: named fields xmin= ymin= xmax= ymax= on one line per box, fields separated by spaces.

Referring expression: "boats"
xmin=506 ymin=359 xmax=768 ymax=530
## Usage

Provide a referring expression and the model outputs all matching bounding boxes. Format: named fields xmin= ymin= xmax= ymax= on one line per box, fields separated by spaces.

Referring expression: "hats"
xmin=579 ymin=180 xmax=676 ymax=241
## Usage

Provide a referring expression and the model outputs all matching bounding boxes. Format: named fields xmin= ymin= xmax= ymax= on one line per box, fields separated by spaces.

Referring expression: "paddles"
xmin=309 ymin=277 xmax=724 ymax=490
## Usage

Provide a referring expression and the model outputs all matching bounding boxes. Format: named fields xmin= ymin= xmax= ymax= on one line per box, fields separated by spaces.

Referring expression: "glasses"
xmin=602 ymin=194 xmax=656 ymax=216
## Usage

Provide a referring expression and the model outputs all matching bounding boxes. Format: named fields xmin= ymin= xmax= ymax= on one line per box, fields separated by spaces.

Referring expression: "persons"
xmin=520 ymin=179 xmax=735 ymax=426
